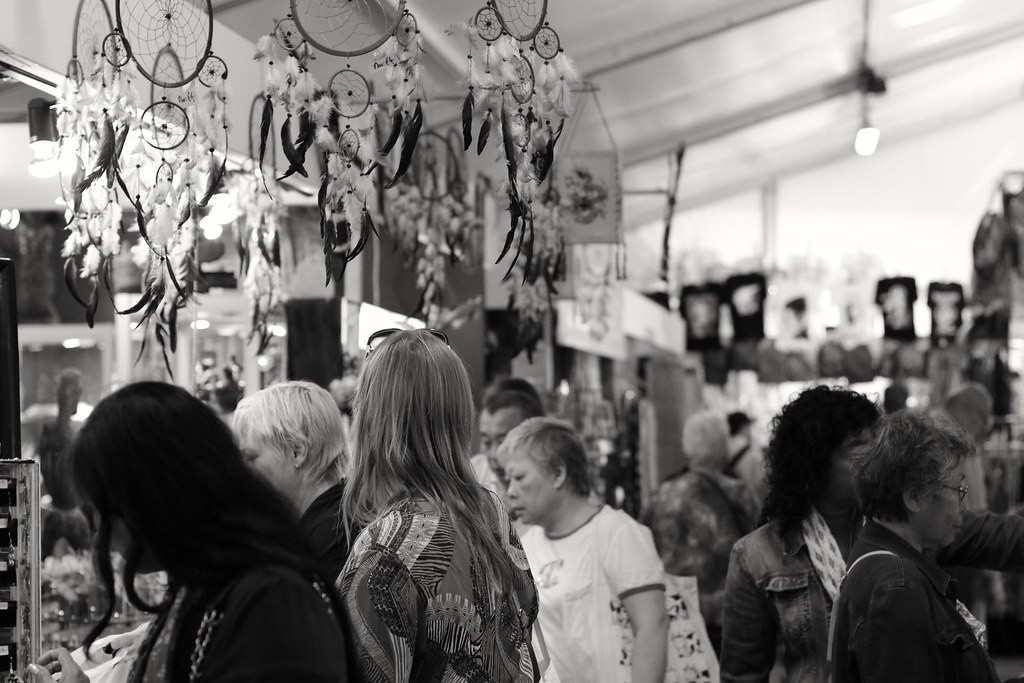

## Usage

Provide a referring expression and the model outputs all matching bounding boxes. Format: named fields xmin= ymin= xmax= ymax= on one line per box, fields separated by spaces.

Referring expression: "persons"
xmin=27 ymin=329 xmax=1024 ymax=683
xmin=32 ymin=370 xmax=90 ymax=559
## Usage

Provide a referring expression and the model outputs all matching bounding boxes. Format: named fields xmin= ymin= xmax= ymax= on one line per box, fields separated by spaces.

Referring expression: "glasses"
xmin=365 ymin=327 xmax=450 ymax=359
xmin=923 ymin=478 xmax=970 ymax=501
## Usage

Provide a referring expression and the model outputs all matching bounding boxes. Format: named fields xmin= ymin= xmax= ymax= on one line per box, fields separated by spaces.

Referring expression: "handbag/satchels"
xmin=605 ymin=572 xmax=723 ymax=683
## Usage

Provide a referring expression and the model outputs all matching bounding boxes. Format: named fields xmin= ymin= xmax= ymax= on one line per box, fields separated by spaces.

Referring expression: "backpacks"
xmin=651 ymin=470 xmax=748 ymax=625
xmin=722 ymin=442 xmax=752 ymax=482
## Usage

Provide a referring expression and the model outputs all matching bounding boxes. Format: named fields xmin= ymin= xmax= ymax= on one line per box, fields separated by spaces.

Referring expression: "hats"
xmin=727 ymin=411 xmax=756 ymax=436
